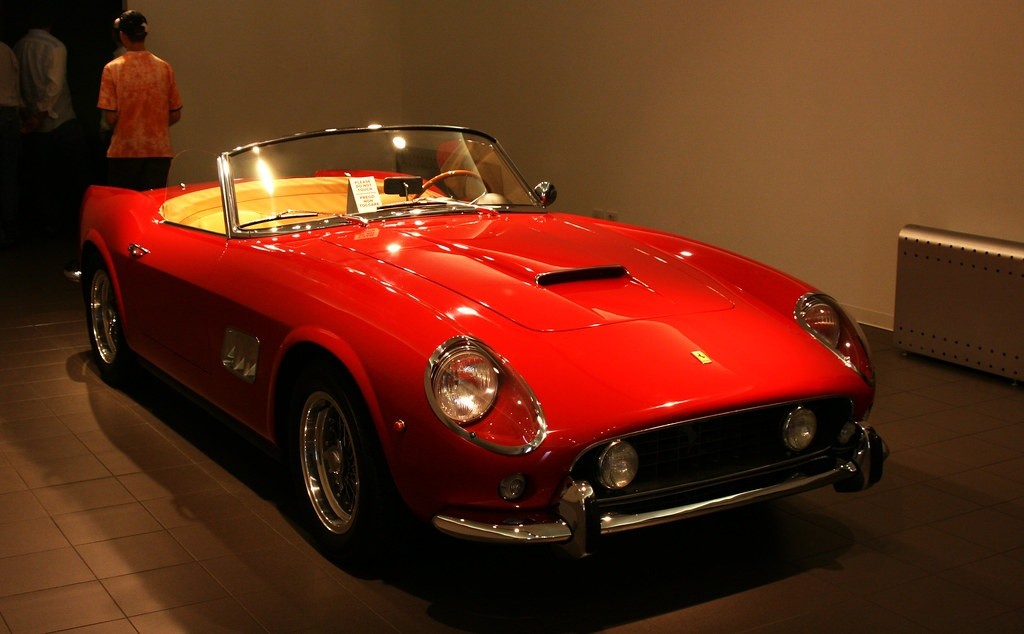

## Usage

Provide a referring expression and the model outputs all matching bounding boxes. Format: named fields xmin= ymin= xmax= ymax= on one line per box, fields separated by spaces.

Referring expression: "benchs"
xmin=161 ymin=176 xmax=443 ymax=235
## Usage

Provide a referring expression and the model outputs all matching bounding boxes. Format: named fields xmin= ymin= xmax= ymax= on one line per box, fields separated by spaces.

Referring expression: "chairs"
xmin=379 ymin=192 xmax=430 ymax=204
xmin=194 ymin=211 xmax=279 ymax=235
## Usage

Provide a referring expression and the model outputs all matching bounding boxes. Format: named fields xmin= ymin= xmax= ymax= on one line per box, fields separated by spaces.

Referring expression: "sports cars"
xmin=77 ymin=125 xmax=892 ymax=560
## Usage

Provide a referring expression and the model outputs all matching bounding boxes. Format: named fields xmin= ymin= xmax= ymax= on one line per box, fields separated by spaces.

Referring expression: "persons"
xmin=0 ymin=8 xmax=79 ymax=253
xmin=97 ymin=10 xmax=185 ymax=192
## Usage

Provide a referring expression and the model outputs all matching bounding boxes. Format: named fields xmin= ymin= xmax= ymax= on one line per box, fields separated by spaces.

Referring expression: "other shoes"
xmin=63 ymin=269 xmax=82 ymax=280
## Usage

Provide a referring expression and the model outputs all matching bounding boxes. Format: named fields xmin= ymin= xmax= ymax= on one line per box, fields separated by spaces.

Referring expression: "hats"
xmin=113 ymin=10 xmax=147 ymax=33
